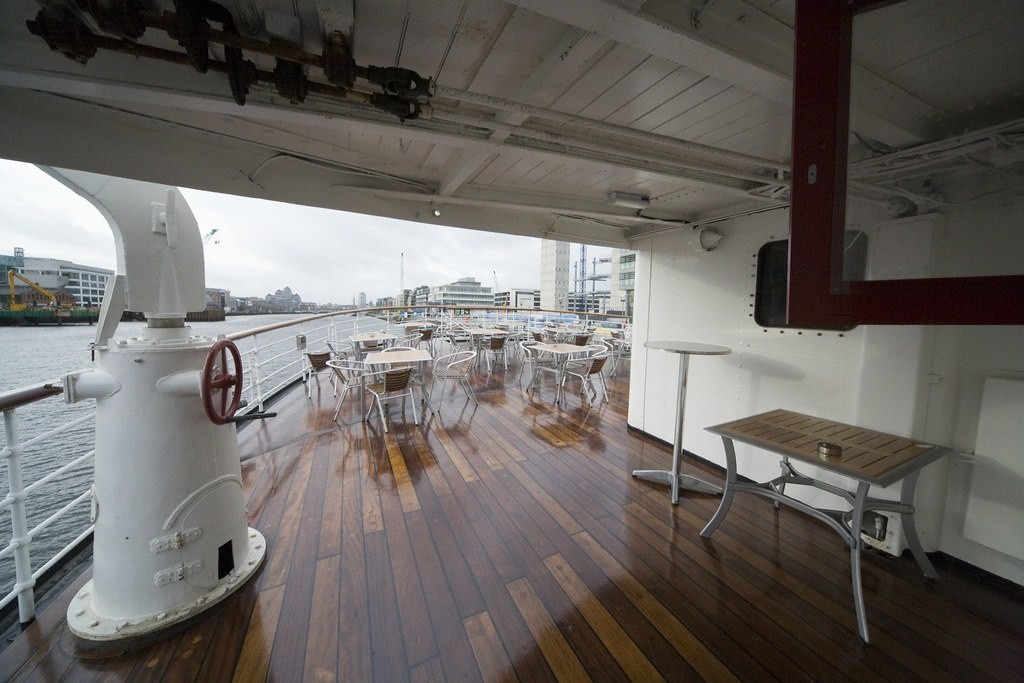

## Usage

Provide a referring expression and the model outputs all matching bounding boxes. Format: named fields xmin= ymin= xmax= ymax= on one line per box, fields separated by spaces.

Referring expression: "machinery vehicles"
xmin=6 ymin=270 xmax=74 ymax=312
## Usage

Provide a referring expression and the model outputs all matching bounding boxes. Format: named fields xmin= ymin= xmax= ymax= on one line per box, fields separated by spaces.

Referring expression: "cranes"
xmin=398 ymin=252 xmax=405 ymax=314
xmin=492 ymin=270 xmax=501 ymax=294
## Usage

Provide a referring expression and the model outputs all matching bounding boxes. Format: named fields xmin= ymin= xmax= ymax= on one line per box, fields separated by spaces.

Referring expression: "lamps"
xmin=606 ymin=192 xmax=650 ymax=209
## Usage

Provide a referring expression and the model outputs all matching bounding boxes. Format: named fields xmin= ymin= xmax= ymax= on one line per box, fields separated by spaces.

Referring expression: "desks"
xmin=700 ymin=408 xmax=955 ymax=646
xmin=469 ymin=328 xmax=511 ymax=368
xmin=394 ymin=322 xmax=437 ymax=354
xmin=364 ymin=348 xmax=435 ymax=416
xmin=498 ymin=322 xmax=528 ymax=326
xmin=348 ymin=333 xmax=396 ymax=375
xmin=303 ymin=318 xmax=632 ymax=434
xmin=595 ymin=327 xmax=613 ymax=335
xmin=543 ymin=328 xmax=573 ymax=343
xmin=525 ymin=344 xmax=597 ymax=405
xmin=632 ymin=340 xmax=732 ymax=504
xmin=559 ymin=322 xmax=578 ymax=326
